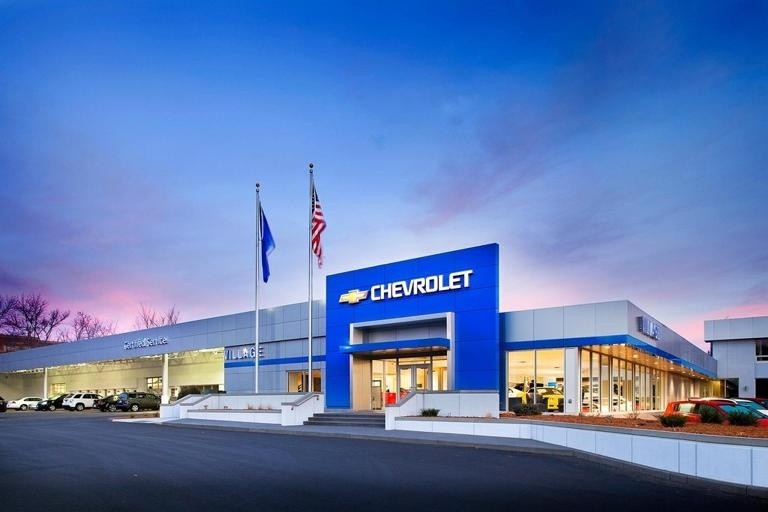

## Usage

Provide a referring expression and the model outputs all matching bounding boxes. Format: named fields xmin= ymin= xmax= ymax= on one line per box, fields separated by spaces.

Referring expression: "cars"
xmin=660 ymin=396 xmax=768 ymax=428
xmin=385 ymin=387 xmax=413 ymax=405
xmin=507 ymin=380 xmax=642 ymax=416
xmin=1 ymin=390 xmax=163 ymax=414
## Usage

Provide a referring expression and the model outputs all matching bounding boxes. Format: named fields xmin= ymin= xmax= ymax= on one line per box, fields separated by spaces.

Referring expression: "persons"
xmin=529 ymin=379 xmax=535 ymax=392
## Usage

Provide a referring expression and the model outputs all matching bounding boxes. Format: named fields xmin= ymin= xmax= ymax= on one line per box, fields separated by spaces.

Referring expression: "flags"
xmin=311 ymin=178 xmax=328 ymax=271
xmin=257 ymin=203 xmax=278 ymax=284
